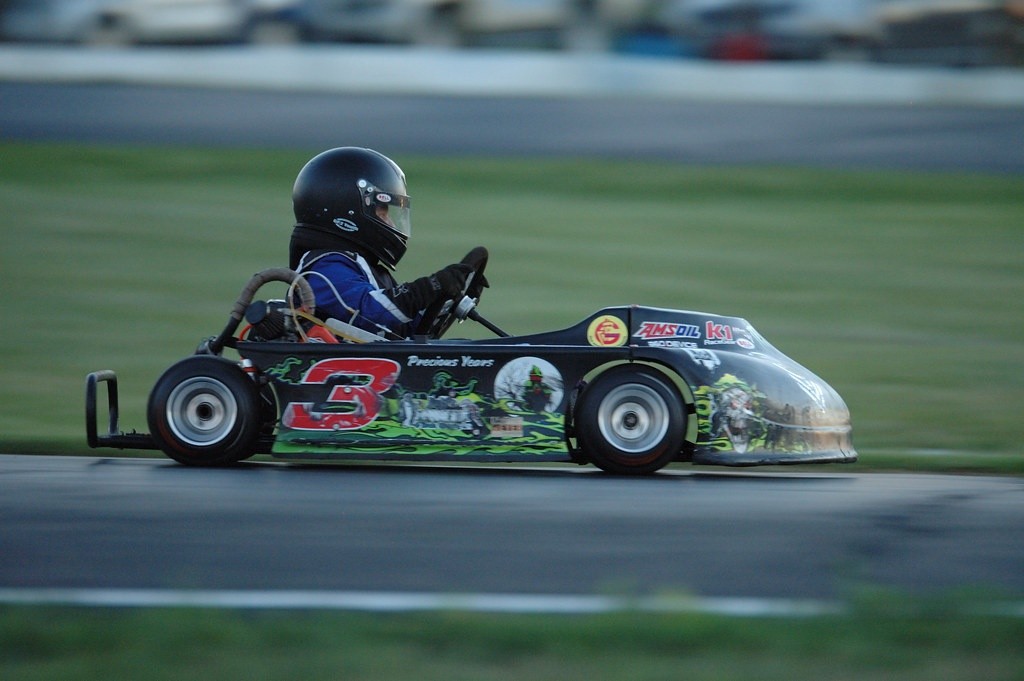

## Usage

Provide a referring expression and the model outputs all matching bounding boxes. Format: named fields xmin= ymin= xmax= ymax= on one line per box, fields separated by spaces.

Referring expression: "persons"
xmin=287 ymin=147 xmax=490 ymax=340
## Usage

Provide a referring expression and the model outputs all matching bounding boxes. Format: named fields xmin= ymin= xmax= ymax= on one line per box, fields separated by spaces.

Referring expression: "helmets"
xmin=293 ymin=147 xmax=411 ymax=266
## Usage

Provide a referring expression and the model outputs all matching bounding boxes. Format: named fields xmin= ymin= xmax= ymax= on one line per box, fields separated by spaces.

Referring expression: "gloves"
xmin=426 ymin=262 xmax=474 ymax=298
